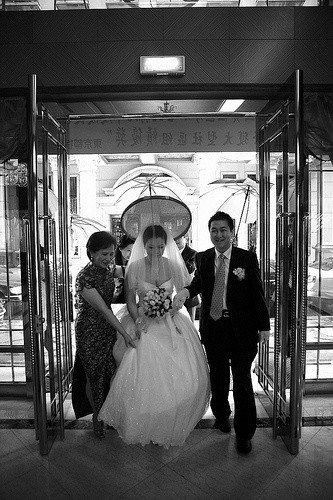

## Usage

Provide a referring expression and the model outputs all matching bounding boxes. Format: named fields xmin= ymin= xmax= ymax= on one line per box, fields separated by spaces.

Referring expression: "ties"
xmin=210 ymin=253 xmax=226 ymax=321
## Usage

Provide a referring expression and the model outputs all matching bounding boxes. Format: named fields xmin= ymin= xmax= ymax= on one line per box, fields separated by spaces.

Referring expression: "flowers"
xmin=141 ymin=285 xmax=182 ymax=334
xmin=232 ymin=267 xmax=245 ymax=280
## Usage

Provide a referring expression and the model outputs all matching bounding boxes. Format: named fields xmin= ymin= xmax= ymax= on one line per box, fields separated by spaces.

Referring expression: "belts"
xmin=222 ymin=309 xmax=231 ymax=318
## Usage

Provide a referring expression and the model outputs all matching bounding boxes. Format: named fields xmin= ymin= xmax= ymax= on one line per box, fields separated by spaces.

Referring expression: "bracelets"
xmin=135 ymin=317 xmax=144 ymax=325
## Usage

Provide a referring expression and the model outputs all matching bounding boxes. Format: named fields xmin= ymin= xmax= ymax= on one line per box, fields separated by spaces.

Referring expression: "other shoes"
xmin=107 ymin=424 xmax=115 ymax=430
xmin=216 ymin=416 xmax=231 ymax=433
xmin=237 ymin=439 xmax=252 ymax=455
xmin=93 ymin=420 xmax=105 ymax=439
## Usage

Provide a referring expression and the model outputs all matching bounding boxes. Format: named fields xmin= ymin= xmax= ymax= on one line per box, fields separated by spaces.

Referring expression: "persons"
xmin=97 ymin=224 xmax=210 ymax=449
xmin=174 ymin=232 xmax=200 ymax=325
xmin=113 ymin=234 xmax=135 ymax=303
xmin=71 ymin=231 xmax=138 ymax=438
xmin=171 ymin=211 xmax=271 ymax=455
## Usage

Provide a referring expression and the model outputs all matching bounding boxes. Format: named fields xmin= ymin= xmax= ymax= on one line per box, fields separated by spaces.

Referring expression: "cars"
xmin=0 ymin=271 xmax=22 ymax=310
xmin=258 ymin=262 xmax=279 ymax=295
xmin=307 ymin=256 xmax=333 ymax=294
